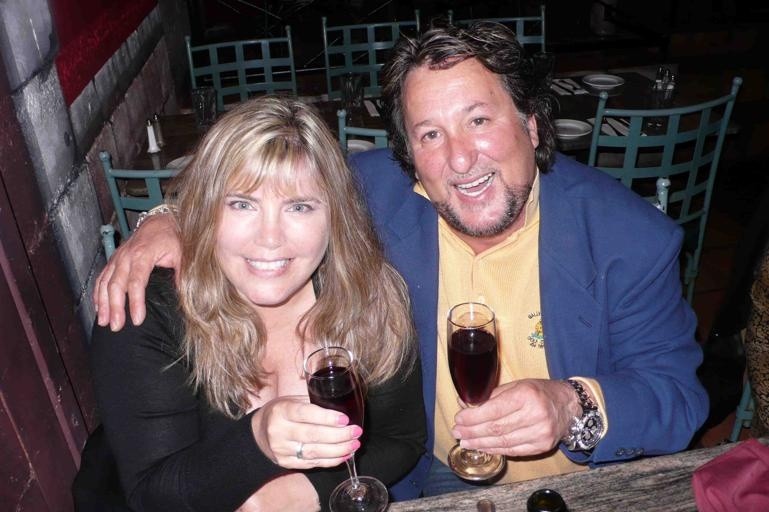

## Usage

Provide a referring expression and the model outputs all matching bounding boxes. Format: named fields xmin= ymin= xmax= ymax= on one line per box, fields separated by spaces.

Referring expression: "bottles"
xmin=152 ymin=115 xmax=167 ymax=149
xmin=144 ymin=119 xmax=161 ymax=155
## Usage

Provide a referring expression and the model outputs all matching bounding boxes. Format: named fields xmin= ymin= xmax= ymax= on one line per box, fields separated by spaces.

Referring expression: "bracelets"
xmin=135 ymin=203 xmax=180 ymax=228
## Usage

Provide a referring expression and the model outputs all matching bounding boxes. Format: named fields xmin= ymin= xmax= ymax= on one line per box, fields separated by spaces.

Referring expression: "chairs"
xmin=184 ymin=26 xmax=298 ymax=109
xmin=337 ymin=109 xmax=390 ymax=157
xmin=100 ymin=150 xmax=189 ymax=241
xmin=448 ymin=6 xmax=546 ymax=59
xmin=701 ymin=375 xmax=753 ymax=446
xmin=322 ymin=9 xmax=420 ymax=101
xmin=588 ymin=76 xmax=741 ymax=303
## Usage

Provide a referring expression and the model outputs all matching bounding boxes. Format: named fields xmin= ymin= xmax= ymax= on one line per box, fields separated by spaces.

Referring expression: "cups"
xmin=192 ymin=85 xmax=217 ymax=126
xmin=526 ymin=489 xmax=568 ymax=512
xmin=346 ymin=116 xmax=367 ymax=139
xmin=339 ymin=71 xmax=364 ymax=116
xmin=200 ymin=126 xmax=214 ymax=139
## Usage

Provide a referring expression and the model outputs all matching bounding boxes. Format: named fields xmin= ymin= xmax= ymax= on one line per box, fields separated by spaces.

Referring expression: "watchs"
xmin=566 ymin=379 xmax=605 ymax=452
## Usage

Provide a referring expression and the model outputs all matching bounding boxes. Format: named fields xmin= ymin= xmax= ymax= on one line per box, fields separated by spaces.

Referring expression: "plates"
xmin=525 ymin=50 xmax=680 ymax=162
xmin=336 ymin=139 xmax=376 ymax=155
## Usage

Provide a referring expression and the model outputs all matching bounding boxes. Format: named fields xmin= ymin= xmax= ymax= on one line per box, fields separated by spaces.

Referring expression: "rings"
xmin=297 ymin=446 xmax=303 ymax=459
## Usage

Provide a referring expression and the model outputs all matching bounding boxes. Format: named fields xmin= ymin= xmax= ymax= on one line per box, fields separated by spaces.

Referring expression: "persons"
xmin=73 ymin=96 xmax=425 ymax=512
xmin=92 ymin=20 xmax=711 ymax=495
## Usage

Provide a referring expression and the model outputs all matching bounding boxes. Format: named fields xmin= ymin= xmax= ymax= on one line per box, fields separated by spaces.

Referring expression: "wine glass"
xmin=444 ymin=300 xmax=511 ymax=485
xmin=302 ymin=345 xmax=389 ymax=511
